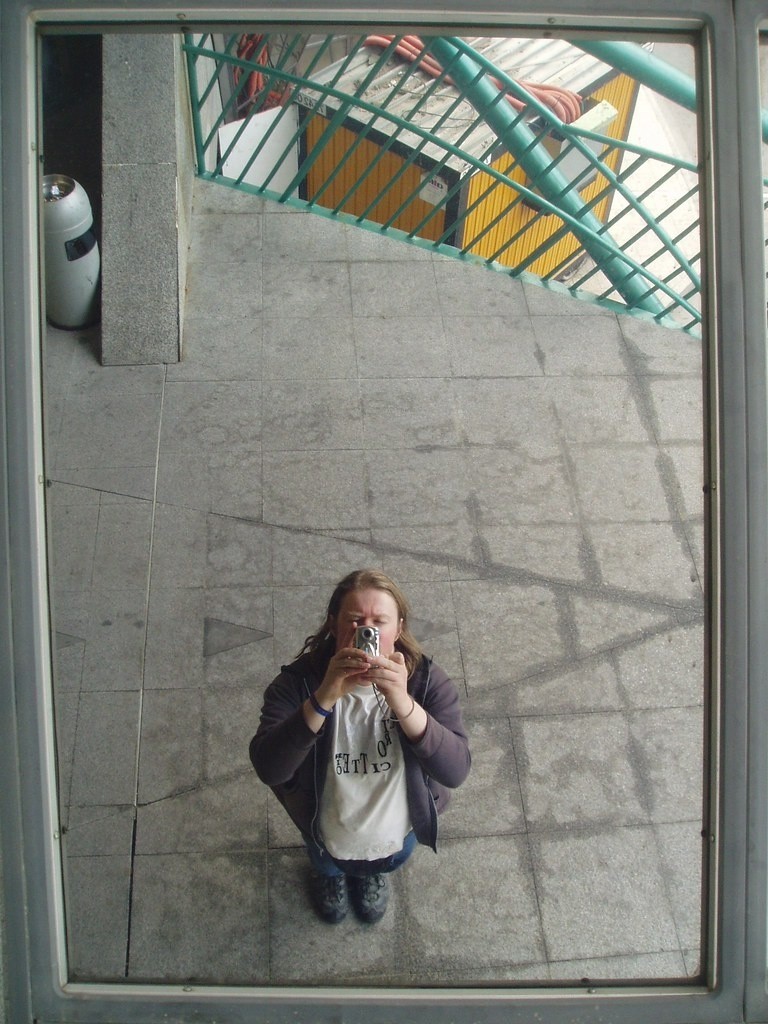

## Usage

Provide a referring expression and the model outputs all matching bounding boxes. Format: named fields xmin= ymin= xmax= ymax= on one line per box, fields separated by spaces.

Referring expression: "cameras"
xmin=356 ymin=625 xmax=380 ymax=668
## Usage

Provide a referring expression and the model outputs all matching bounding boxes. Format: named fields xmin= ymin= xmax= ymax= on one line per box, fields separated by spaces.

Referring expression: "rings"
xmin=344 ymin=671 xmax=349 ymax=678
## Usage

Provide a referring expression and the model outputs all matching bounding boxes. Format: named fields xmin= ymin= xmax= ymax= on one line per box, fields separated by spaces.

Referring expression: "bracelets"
xmin=310 ymin=694 xmax=333 ymax=717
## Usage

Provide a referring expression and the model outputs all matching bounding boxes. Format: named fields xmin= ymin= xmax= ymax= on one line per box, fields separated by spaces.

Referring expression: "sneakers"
xmin=352 ymin=873 xmax=389 ymax=923
xmin=315 ymin=874 xmax=349 ymax=922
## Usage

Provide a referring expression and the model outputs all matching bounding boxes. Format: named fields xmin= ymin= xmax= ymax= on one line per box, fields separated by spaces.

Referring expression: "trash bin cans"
xmin=41 ymin=173 xmax=101 ymax=329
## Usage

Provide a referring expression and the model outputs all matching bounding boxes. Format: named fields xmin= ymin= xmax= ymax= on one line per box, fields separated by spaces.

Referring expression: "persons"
xmin=249 ymin=567 xmax=471 ymax=924
